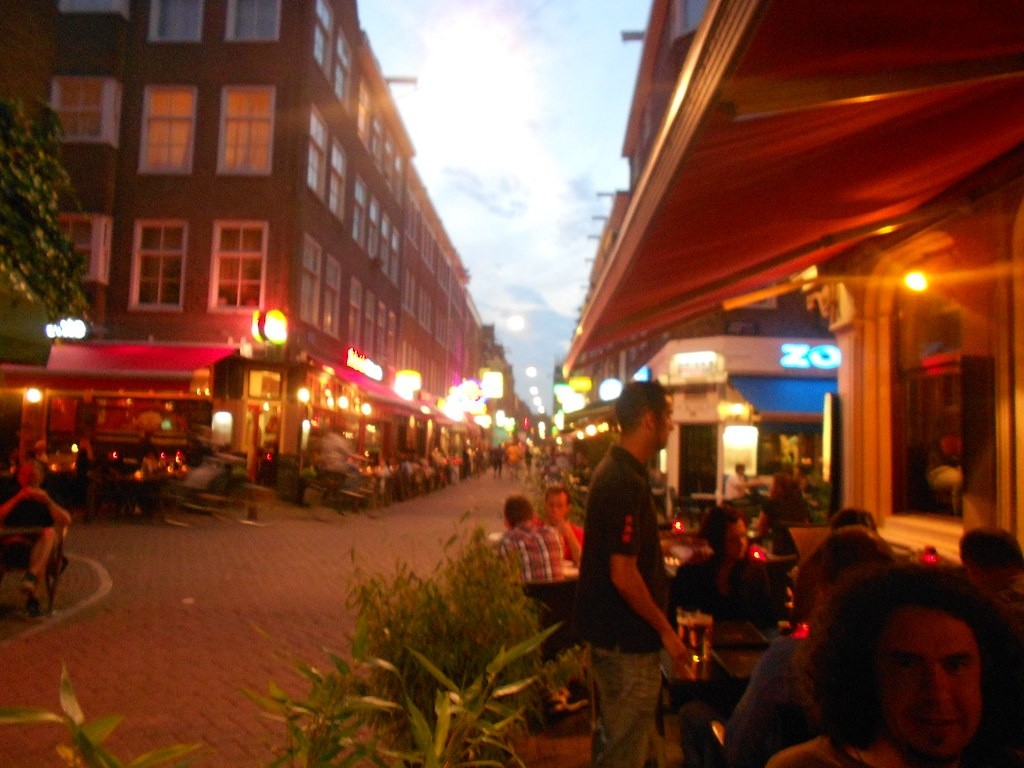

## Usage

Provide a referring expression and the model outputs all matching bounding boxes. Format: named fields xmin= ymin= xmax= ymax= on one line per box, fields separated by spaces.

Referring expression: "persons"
xmin=498 ymin=496 xmax=562 ymax=579
xmin=737 ymin=493 xmax=798 ymax=555
xmin=765 ymin=556 xmax=1024 ymax=768
xmin=324 ymin=423 xmax=368 ymax=512
xmin=17 ymin=440 xmax=51 ymax=487
xmin=725 ymin=525 xmax=896 ymax=768
xmin=369 ymin=446 xmax=470 ymax=478
xmin=962 ymin=527 xmax=1024 ymax=636
xmin=575 ymin=382 xmax=687 ymax=768
xmin=135 ymin=430 xmax=159 ymax=459
xmin=793 ymin=508 xmax=876 ymax=621
xmin=186 ymin=437 xmax=203 ymax=467
xmin=76 ymin=437 xmax=94 ymax=472
xmin=670 ymin=503 xmax=794 ymax=636
xmin=535 ymin=487 xmax=584 ymax=562
xmin=769 ymin=461 xmax=812 ymax=504
xmin=491 ymin=442 xmax=523 ymax=481
xmin=725 ymin=464 xmax=747 ymax=499
xmin=0 ymin=459 xmax=71 ymax=614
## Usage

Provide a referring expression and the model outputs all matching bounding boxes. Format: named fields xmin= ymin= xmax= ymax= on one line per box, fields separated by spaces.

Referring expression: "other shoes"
xmin=27 ymin=597 xmax=41 ymax=616
xmin=546 ymin=688 xmax=588 ymax=715
xmin=21 ymin=570 xmax=37 ymax=592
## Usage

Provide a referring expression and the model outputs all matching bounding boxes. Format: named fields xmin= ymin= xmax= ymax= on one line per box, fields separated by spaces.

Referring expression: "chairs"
xmin=527 ymin=578 xmax=597 ymax=729
xmin=670 ymin=488 xmax=694 ymax=528
xmin=10 ymin=526 xmax=69 ymax=611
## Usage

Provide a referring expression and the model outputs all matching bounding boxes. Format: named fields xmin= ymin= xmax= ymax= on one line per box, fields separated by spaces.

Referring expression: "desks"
xmin=690 ymin=491 xmax=715 ymax=517
xmin=654 ymin=626 xmax=787 ymax=734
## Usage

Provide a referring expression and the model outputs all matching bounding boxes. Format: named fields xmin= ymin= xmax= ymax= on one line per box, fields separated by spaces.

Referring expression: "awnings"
xmin=0 ymin=338 xmax=236 ymax=394
xmin=310 ymin=353 xmax=435 ymax=421
xmin=727 ymin=377 xmax=838 ymax=424
xmin=562 ymin=0 xmax=1024 ymax=381
